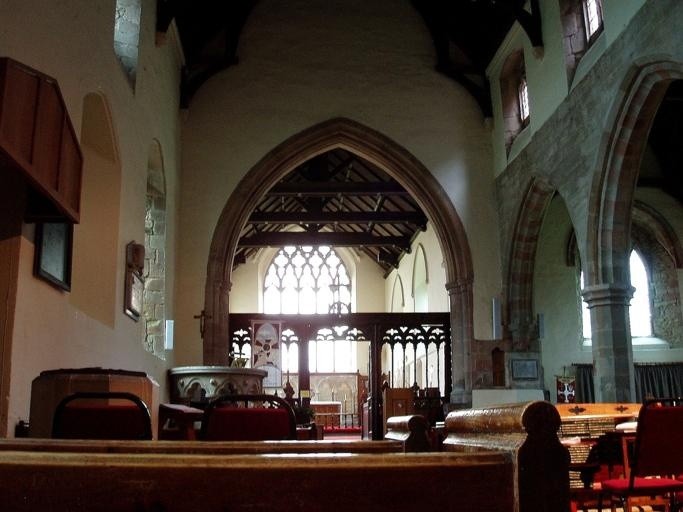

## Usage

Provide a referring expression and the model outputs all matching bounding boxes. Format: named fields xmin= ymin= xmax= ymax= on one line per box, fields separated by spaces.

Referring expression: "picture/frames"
xmin=33 ymin=221 xmax=73 ymax=294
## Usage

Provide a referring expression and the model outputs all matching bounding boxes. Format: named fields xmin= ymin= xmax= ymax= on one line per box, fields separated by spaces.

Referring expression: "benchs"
xmin=1 ymin=432 xmax=406 ymax=455
xmin=0 ymin=450 xmax=515 ymax=510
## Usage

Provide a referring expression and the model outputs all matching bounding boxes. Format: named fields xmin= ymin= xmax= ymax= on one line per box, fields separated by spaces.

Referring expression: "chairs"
xmin=566 ymin=395 xmax=681 ymax=512
xmin=48 ymin=384 xmax=298 ymax=442
